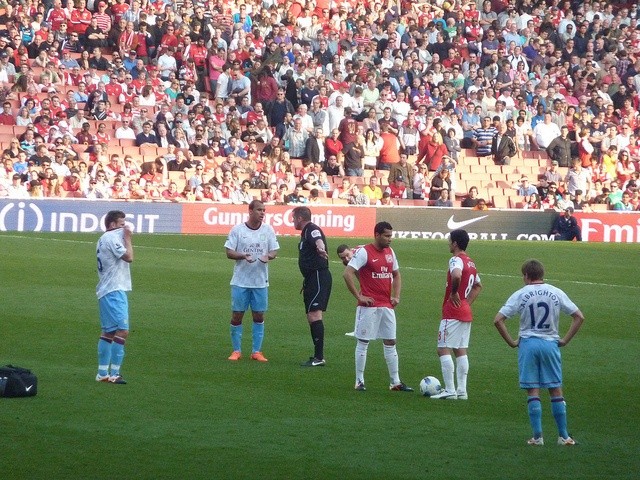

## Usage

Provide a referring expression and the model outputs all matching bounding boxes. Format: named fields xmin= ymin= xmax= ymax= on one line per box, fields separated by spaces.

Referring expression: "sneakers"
xmin=558 ymin=435 xmax=576 ymax=445
xmin=431 ymin=390 xmax=458 ymax=399
xmin=108 ymin=376 xmax=128 ymax=384
xmin=301 ymin=357 xmax=327 ymax=366
xmin=458 ymin=391 xmax=469 ymax=400
xmin=346 ymin=331 xmax=355 ymax=337
xmin=355 ymin=383 xmax=366 ymax=390
xmin=390 ymin=382 xmax=415 ymax=392
xmin=96 ymin=373 xmax=111 ymax=382
xmin=229 ymin=350 xmax=242 ymax=360
xmin=251 ymin=350 xmax=269 ymax=361
xmin=529 ymin=436 xmax=545 ymax=446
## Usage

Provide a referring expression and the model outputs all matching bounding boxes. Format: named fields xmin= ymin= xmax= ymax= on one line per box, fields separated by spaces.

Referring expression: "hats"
xmin=396 ymin=176 xmax=406 ymax=182
xmin=564 ymin=206 xmax=574 ymax=212
xmin=576 ymin=189 xmax=583 ymax=195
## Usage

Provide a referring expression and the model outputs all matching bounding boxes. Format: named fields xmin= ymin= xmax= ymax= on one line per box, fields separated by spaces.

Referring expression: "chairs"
xmin=289 ymin=145 xmax=637 ymax=209
xmin=0 ymin=35 xmax=287 ymax=204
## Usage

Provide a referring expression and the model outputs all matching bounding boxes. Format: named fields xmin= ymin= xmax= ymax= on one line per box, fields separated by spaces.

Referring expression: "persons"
xmin=494 ymin=261 xmax=584 ymax=445
xmin=551 ymin=207 xmax=581 ymax=241
xmin=292 ymin=206 xmax=332 ymax=366
xmin=1 ymin=1 xmax=640 ymax=211
xmin=343 ymin=222 xmax=415 ymax=392
xmin=96 ymin=211 xmax=132 ymax=384
xmin=430 ymin=229 xmax=481 ymax=399
xmin=337 ymin=245 xmax=365 ymax=336
xmin=224 ymin=200 xmax=280 ymax=362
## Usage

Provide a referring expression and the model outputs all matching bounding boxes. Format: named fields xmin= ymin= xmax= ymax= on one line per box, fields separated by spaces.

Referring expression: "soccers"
xmin=419 ymin=375 xmax=443 ymax=396
